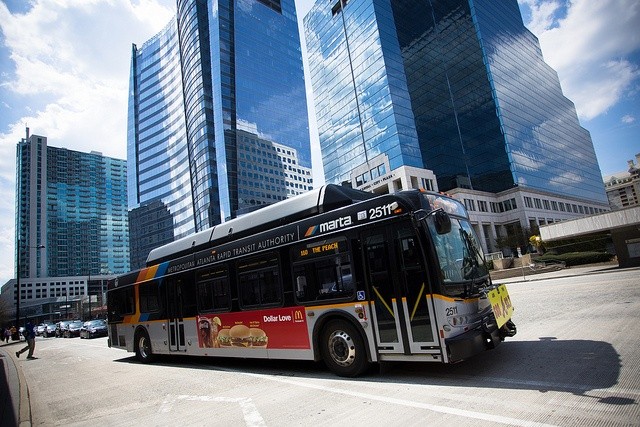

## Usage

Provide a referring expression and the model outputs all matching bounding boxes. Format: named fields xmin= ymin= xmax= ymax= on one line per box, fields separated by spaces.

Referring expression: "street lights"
xmin=16 ymin=239 xmax=45 ymax=339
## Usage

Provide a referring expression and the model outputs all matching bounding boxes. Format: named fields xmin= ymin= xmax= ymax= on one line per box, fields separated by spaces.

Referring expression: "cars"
xmin=63 ymin=322 xmax=84 ymax=338
xmin=79 ymin=319 xmax=108 ymax=339
xmin=43 ymin=324 xmax=56 ymax=337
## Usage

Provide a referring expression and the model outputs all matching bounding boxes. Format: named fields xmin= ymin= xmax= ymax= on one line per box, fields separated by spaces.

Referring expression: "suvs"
xmin=55 ymin=323 xmax=60 ymax=338
xmin=59 ymin=320 xmax=71 ymax=338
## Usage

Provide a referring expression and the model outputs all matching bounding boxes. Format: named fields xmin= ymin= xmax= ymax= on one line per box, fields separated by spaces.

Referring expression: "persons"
xmin=16 ymin=318 xmax=35 ymax=360
xmin=9 ymin=326 xmax=17 ymax=340
xmin=4 ymin=326 xmax=11 ymax=343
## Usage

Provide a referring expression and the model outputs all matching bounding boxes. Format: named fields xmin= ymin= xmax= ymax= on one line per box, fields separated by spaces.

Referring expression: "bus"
xmin=105 ymin=182 xmax=518 ymax=378
